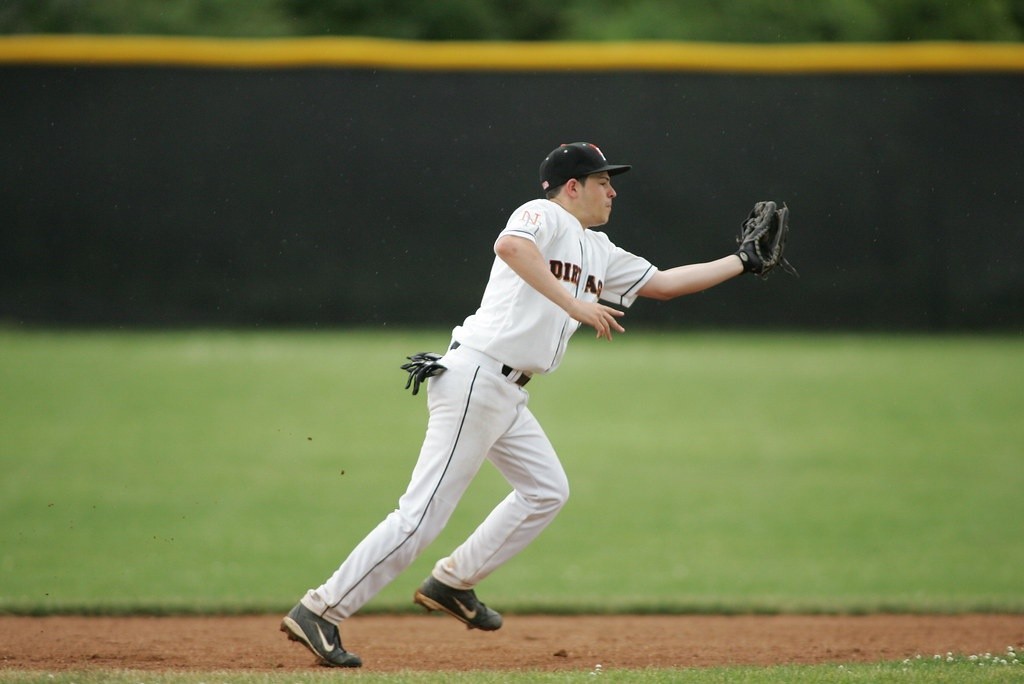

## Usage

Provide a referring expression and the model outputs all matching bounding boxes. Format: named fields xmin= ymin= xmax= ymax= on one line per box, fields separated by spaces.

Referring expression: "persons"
xmin=279 ymin=139 xmax=790 ymax=671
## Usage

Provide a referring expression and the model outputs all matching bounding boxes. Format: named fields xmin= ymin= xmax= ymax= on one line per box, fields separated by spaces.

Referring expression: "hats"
xmin=539 ymin=142 xmax=633 ymax=194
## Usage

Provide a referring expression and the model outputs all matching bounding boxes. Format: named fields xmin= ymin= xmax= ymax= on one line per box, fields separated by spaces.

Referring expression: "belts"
xmin=451 ymin=342 xmax=530 ymax=387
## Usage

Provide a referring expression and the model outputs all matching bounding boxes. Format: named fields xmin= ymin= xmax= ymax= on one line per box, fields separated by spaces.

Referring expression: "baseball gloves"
xmin=733 ymin=200 xmax=802 ymax=282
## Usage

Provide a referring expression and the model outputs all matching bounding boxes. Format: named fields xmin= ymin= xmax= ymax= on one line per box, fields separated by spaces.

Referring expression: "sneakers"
xmin=414 ymin=575 xmax=502 ymax=632
xmin=280 ymin=604 xmax=362 ymax=669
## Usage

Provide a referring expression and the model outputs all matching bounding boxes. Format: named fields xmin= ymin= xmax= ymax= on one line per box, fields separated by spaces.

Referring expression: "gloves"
xmin=402 ymin=352 xmax=446 ymax=396
